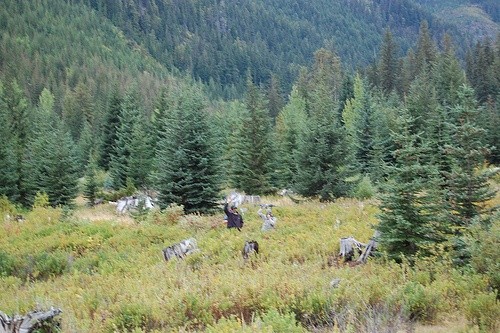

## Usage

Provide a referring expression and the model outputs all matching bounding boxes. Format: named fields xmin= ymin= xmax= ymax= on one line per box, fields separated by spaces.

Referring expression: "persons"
xmin=223 ymin=195 xmax=243 ymax=235
